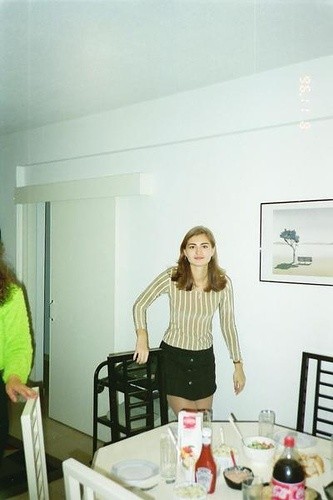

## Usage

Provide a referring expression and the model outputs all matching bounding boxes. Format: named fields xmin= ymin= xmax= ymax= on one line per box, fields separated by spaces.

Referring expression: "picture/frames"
xmin=259 ymin=199 xmax=333 ymax=286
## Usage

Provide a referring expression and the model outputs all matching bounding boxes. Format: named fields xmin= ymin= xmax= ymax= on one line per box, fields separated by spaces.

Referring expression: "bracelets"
xmin=233 ymin=359 xmax=243 ymax=363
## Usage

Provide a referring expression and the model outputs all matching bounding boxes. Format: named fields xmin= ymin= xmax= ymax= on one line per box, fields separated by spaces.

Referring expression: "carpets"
xmin=0 ymin=448 xmax=63 ymax=500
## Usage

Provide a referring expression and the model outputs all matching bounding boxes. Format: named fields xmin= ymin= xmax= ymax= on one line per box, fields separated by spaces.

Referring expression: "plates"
xmin=112 ymin=458 xmax=159 ymax=481
xmin=277 ymin=431 xmax=317 ymax=456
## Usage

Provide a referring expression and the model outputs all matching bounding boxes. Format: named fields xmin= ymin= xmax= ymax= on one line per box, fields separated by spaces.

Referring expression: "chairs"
xmin=62 ymin=457 xmax=143 ymax=500
xmin=107 ymin=349 xmax=178 ymax=444
xmin=295 ymin=351 xmax=333 ymax=441
xmin=20 ymin=386 xmax=49 ymax=500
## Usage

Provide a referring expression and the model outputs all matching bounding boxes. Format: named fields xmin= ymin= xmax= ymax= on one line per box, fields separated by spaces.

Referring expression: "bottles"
xmin=194 ymin=428 xmax=217 ymax=496
xmin=270 ymin=436 xmax=305 ymax=500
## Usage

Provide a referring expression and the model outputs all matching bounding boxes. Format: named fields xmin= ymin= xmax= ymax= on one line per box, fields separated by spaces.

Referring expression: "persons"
xmin=0 ymin=230 xmax=39 ymax=454
xmin=133 ymin=225 xmax=246 ymax=421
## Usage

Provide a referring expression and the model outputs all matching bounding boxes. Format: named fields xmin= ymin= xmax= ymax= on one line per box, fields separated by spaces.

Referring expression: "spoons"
xmin=306 ymin=477 xmax=328 ymax=500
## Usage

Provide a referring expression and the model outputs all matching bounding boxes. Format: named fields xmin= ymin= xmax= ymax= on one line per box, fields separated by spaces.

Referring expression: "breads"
xmin=296 ymin=454 xmax=326 ymax=478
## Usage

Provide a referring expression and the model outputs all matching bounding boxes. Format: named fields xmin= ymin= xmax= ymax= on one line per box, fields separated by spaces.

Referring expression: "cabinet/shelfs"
xmin=92 ymin=359 xmax=177 ymax=458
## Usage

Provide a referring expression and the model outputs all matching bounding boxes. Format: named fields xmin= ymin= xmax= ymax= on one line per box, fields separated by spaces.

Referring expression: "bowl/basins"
xmin=243 ymin=436 xmax=276 ymax=461
xmin=213 ymin=446 xmax=241 ymax=470
xmin=223 ymin=466 xmax=254 ymax=490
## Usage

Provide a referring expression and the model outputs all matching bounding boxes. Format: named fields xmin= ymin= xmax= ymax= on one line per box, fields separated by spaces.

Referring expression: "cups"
xmin=242 ymin=476 xmax=264 ymax=500
xmin=159 ymin=439 xmax=177 ymax=484
xmin=258 ymin=410 xmax=275 ymax=437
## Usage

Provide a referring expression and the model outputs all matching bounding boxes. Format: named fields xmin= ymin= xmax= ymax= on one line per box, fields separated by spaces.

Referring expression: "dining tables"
xmin=91 ymin=422 xmax=333 ymax=500
